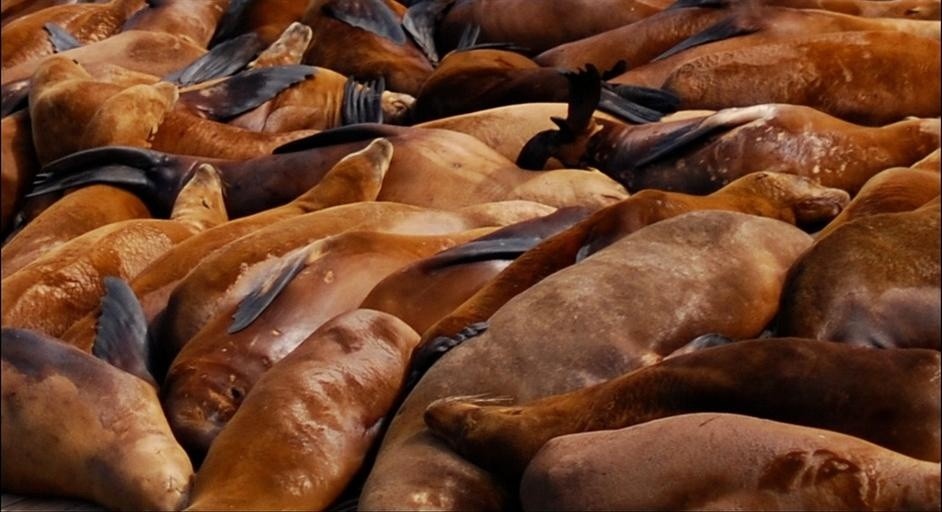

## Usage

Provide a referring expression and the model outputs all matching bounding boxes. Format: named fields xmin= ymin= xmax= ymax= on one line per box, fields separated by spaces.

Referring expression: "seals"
xmin=0 ymin=0 xmax=941 ymax=511
